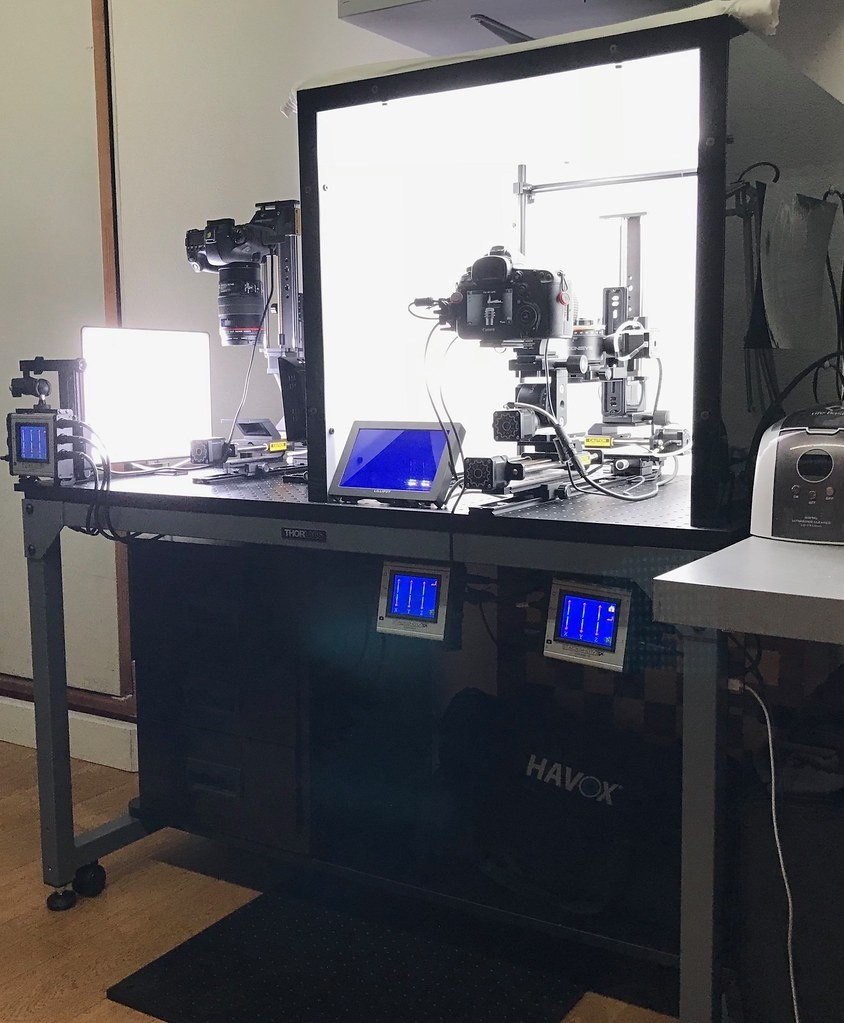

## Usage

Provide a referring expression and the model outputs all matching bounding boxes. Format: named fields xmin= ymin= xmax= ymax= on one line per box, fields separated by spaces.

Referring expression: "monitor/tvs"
xmin=328 ymin=421 xmax=466 ymax=508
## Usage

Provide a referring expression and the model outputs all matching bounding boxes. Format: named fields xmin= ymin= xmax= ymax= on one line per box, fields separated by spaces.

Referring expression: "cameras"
xmin=443 ymin=245 xmax=579 ymax=340
xmin=185 ymin=225 xmax=276 ymax=347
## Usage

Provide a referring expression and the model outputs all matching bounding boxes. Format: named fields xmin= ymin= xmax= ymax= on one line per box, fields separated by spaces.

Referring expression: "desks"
xmin=13 ymin=471 xmax=844 ymax=1023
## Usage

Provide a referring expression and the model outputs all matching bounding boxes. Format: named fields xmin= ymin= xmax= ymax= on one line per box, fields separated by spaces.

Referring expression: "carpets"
xmin=107 ymin=873 xmax=590 ymax=1023
